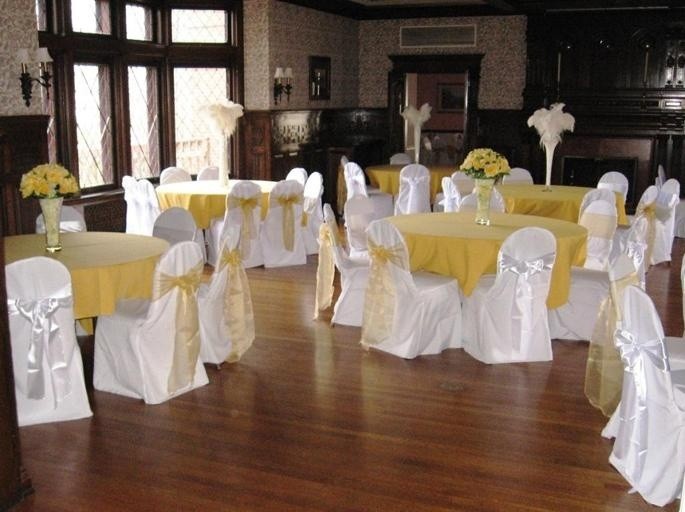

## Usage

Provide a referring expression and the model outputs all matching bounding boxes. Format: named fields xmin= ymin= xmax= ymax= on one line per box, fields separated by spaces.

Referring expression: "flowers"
xmin=457 ymin=143 xmax=514 ymax=180
xmin=20 ymin=164 xmax=78 ymax=199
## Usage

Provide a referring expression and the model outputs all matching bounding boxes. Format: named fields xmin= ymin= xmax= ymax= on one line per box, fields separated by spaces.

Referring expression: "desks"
xmin=1 ymin=232 xmax=169 ymax=338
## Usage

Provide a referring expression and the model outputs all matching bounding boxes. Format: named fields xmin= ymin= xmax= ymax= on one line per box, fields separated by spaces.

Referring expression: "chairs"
xmin=195 ymin=225 xmax=255 ymax=366
xmin=584 ymin=253 xmax=685 ymax=412
xmin=5 ymin=256 xmax=93 ymax=427
xmin=94 ymin=241 xmax=210 ymax=406
xmin=325 ymin=153 xmax=685 ymax=364
xmin=602 ymin=286 xmax=685 ymax=512
xmin=122 ymin=161 xmax=326 ymax=269
xmin=36 ymin=207 xmax=84 ymax=233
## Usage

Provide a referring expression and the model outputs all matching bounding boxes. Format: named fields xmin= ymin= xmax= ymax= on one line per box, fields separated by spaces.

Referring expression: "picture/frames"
xmin=435 ymin=82 xmax=464 ymax=114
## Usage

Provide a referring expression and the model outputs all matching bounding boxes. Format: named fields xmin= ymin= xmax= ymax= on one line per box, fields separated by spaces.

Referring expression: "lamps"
xmin=273 ymin=64 xmax=293 ymax=105
xmin=14 ymin=45 xmax=55 ymax=107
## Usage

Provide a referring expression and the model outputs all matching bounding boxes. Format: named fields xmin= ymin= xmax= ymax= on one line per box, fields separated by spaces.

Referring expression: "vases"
xmin=39 ymin=199 xmax=63 ymax=250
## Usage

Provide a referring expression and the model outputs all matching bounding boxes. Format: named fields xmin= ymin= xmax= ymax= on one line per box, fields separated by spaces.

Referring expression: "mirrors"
xmin=308 ymin=54 xmax=331 ymax=100
